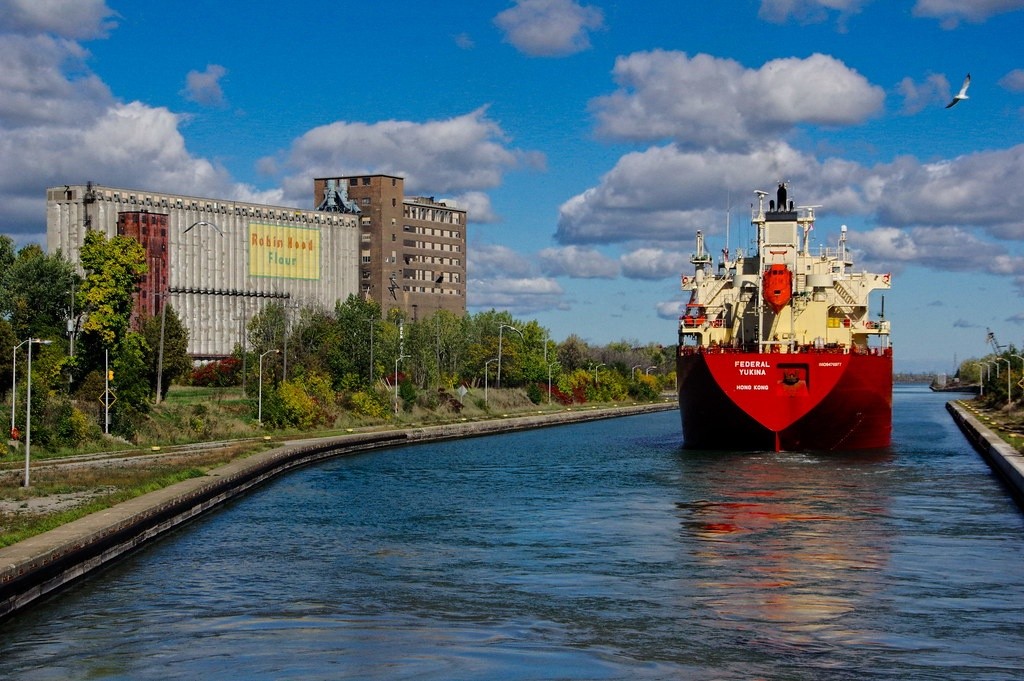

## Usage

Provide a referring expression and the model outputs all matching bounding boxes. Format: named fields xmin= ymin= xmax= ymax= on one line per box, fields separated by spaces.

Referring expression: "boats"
xmin=676 ymin=181 xmax=894 ymax=454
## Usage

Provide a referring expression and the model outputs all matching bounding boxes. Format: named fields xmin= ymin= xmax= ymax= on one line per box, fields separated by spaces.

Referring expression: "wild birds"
xmin=945 ymin=73 xmax=971 ymax=109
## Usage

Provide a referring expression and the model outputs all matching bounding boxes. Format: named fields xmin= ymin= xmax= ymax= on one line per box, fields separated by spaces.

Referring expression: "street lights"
xmin=632 ymin=365 xmax=658 ymax=381
xmin=394 ymin=355 xmax=412 ymax=414
xmin=485 ymin=359 xmax=498 ymax=408
xmin=12 ymin=336 xmax=55 ymax=488
xmin=596 ymin=364 xmax=606 ymax=385
xmin=972 ymin=357 xmax=1011 ymax=419
xmin=259 ymin=349 xmax=280 ymax=423
xmin=548 ymin=361 xmax=561 ymax=406
